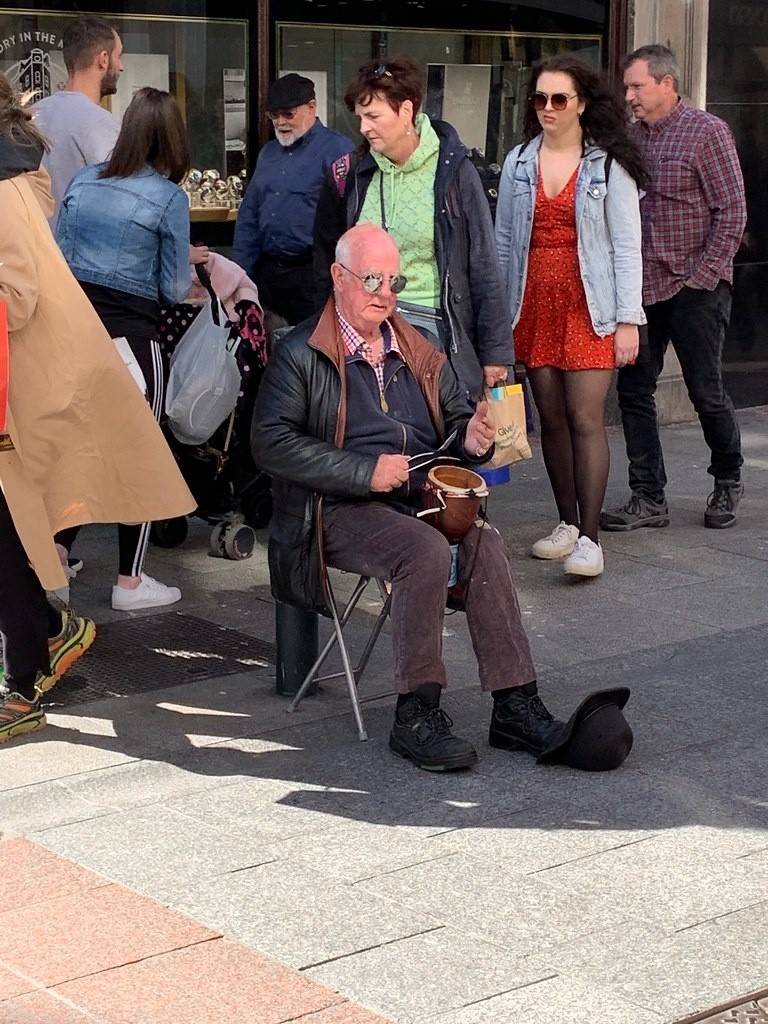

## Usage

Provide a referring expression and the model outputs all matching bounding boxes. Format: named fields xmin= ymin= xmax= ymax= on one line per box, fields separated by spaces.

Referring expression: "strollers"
xmin=154 ymin=262 xmax=276 ymax=561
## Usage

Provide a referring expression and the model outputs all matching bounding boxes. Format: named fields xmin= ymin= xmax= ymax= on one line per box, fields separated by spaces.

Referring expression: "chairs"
xmin=283 ymin=563 xmax=395 ymax=743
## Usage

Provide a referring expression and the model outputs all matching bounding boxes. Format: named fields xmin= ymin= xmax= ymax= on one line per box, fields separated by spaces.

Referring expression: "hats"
xmin=533 ymin=687 xmax=634 ymax=772
xmin=265 ymin=73 xmax=316 ymax=109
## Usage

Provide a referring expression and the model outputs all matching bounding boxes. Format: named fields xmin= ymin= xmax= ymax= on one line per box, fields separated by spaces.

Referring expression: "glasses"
xmin=356 ymin=62 xmax=395 ymax=79
xmin=528 ymin=91 xmax=578 ymax=111
xmin=340 ymin=263 xmax=408 ymax=294
xmin=265 ymin=108 xmax=297 ymax=121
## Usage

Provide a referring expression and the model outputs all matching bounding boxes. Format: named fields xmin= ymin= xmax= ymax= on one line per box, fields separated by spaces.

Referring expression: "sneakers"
xmin=388 ymin=696 xmax=478 ymax=771
xmin=0 ymin=676 xmax=48 ymax=744
xmin=33 ymin=608 xmax=97 ymax=697
xmin=703 ymin=475 xmax=744 ymax=529
xmin=46 ymin=565 xmax=76 ymax=607
xmin=489 ymin=685 xmax=565 ymax=760
xmin=601 ymin=490 xmax=670 ymax=531
xmin=564 ymin=535 xmax=604 ymax=576
xmin=111 ymin=571 xmax=182 ymax=611
xmin=532 ymin=520 xmax=580 ymax=558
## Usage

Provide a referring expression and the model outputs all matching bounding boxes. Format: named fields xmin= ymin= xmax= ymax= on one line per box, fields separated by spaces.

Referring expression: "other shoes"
xmin=68 ymin=557 xmax=85 ymax=572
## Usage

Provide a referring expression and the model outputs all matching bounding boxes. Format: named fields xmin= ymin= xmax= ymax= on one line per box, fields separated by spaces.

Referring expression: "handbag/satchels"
xmin=164 ymin=296 xmax=242 ymax=446
xmin=481 ymin=379 xmax=532 ymax=469
xmin=0 ymin=297 xmax=10 ymax=433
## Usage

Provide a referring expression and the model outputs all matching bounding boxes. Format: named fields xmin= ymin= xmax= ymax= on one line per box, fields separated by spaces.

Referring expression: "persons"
xmin=0 ymin=73 xmax=201 ymax=748
xmin=493 ymin=52 xmax=647 ymax=579
xmin=599 ymin=44 xmax=746 ymax=532
xmin=229 ymin=73 xmax=356 ymax=359
xmin=309 ymin=53 xmax=515 ymax=407
xmin=54 ymin=86 xmax=211 ymax=613
xmin=23 ymin=14 xmax=124 ymax=224
xmin=250 ymin=224 xmax=568 ymax=775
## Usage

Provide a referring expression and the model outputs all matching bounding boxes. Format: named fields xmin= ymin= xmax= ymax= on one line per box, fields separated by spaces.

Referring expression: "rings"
xmin=475 ymin=447 xmax=483 ymax=456
xmin=499 ymin=376 xmax=503 ymax=380
xmin=623 ymin=360 xmax=627 ymax=365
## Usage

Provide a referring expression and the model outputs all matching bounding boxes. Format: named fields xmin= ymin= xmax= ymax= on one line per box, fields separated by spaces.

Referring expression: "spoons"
xmin=407 ymin=456 xmax=461 ymax=472
xmin=406 ymin=429 xmax=457 ymax=463
